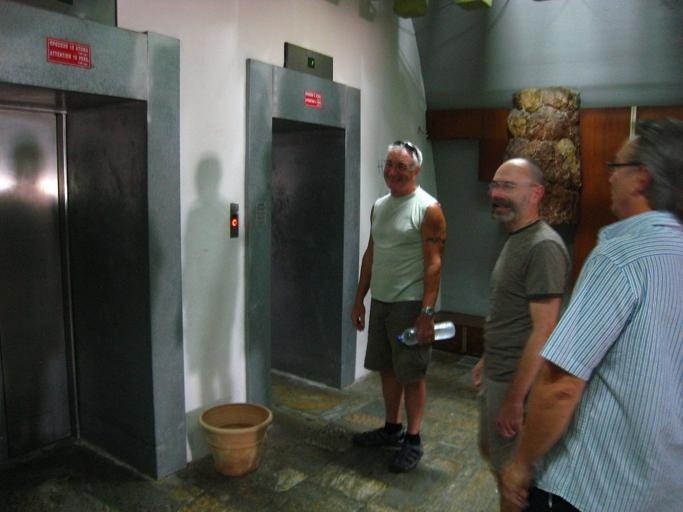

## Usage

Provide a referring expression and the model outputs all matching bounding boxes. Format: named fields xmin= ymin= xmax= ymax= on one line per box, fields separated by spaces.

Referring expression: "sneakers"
xmin=391 ymin=443 xmax=423 ymax=472
xmin=353 ymin=427 xmax=404 ymax=450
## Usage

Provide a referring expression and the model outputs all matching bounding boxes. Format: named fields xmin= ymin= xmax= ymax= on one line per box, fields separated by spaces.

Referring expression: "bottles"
xmin=396 ymin=321 xmax=456 ymax=346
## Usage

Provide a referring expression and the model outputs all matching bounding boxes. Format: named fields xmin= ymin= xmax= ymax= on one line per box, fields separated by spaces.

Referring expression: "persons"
xmin=498 ymin=113 xmax=683 ymax=512
xmin=471 ymin=156 xmax=577 ymax=512
xmin=350 ymin=138 xmax=448 ymax=474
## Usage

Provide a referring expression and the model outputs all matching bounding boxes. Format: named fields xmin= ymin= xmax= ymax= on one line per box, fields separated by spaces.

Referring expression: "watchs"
xmin=419 ymin=305 xmax=435 ymax=317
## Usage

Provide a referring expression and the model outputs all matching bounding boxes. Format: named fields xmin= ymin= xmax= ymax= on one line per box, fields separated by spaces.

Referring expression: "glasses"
xmin=608 ymin=159 xmax=643 ymax=173
xmin=395 ymin=139 xmax=418 ymax=158
